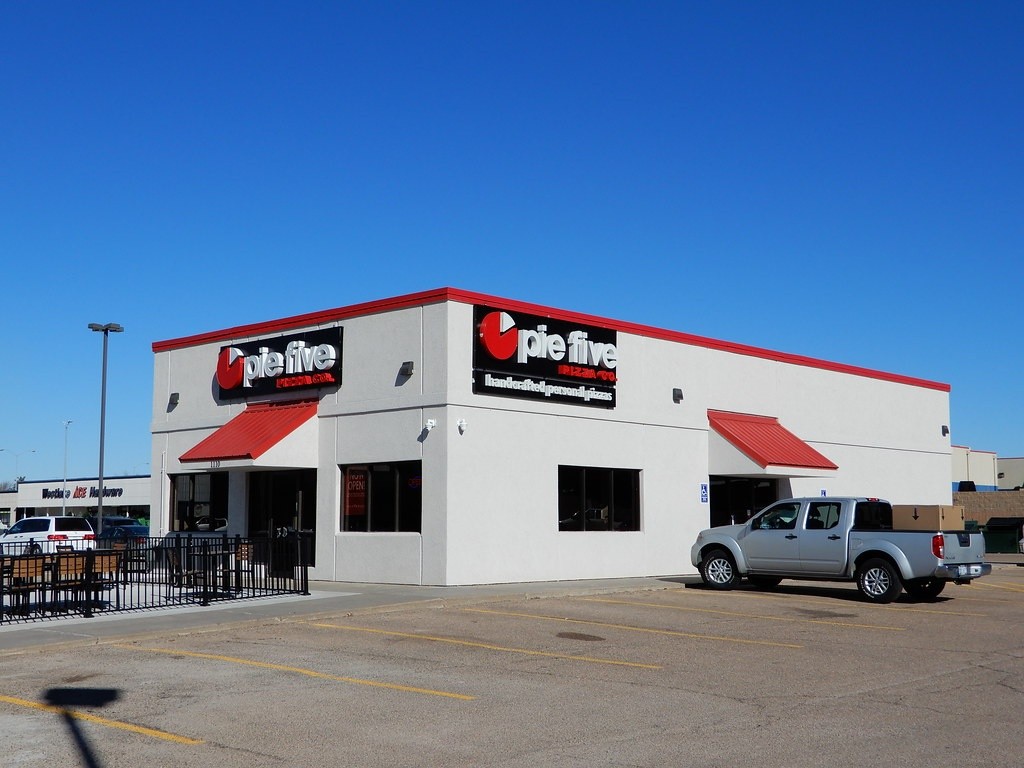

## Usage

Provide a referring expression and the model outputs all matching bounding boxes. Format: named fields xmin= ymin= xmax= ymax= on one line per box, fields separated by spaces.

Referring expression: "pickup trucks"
xmin=688 ymin=495 xmax=992 ymax=604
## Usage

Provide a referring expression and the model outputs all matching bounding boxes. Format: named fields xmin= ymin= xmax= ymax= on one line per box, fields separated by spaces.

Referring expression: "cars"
xmin=99 ymin=526 xmax=149 ymax=557
xmin=194 ymin=516 xmax=210 ymax=531
xmin=86 ymin=514 xmax=142 ymax=536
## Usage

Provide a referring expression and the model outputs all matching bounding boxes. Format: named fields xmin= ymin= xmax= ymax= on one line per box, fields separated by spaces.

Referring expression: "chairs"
xmin=803 ymin=506 xmax=824 ymax=529
xmin=163 ymin=547 xmax=202 ymax=600
xmin=47 ymin=553 xmax=86 ymax=613
xmin=828 ymin=506 xmax=840 ymax=528
xmin=85 ymin=548 xmax=123 ymax=610
xmin=221 ymin=541 xmax=255 ymax=597
xmin=4 ymin=557 xmax=45 ymax=618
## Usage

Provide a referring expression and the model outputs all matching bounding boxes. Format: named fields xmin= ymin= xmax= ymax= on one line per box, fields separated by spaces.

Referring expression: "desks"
xmin=189 ymin=550 xmax=236 ymax=590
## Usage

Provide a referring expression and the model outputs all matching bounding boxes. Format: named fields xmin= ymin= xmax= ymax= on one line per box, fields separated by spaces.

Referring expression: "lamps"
xmin=400 ymin=361 xmax=414 ymax=376
xmin=169 ymin=393 xmax=179 ymax=405
xmin=942 ymin=425 xmax=949 ymax=435
xmin=673 ymin=387 xmax=683 ymax=400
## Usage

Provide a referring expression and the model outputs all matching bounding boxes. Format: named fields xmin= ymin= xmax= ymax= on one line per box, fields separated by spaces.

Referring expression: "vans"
xmin=0 ymin=515 xmax=97 ymax=555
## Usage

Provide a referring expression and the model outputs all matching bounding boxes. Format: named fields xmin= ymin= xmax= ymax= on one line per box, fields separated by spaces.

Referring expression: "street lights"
xmin=87 ymin=321 xmax=125 ymax=537
xmin=60 ymin=419 xmax=74 ymax=515
xmin=0 ymin=448 xmax=37 ymax=493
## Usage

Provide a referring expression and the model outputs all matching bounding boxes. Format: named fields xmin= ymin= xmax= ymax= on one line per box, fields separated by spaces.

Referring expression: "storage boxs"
xmin=893 ymin=506 xmax=964 ymax=531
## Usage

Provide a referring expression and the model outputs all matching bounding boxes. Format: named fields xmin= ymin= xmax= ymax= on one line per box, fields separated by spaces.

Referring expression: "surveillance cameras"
xmin=425 ymin=423 xmax=432 ymax=432
xmin=460 ymin=419 xmax=468 ymax=432
